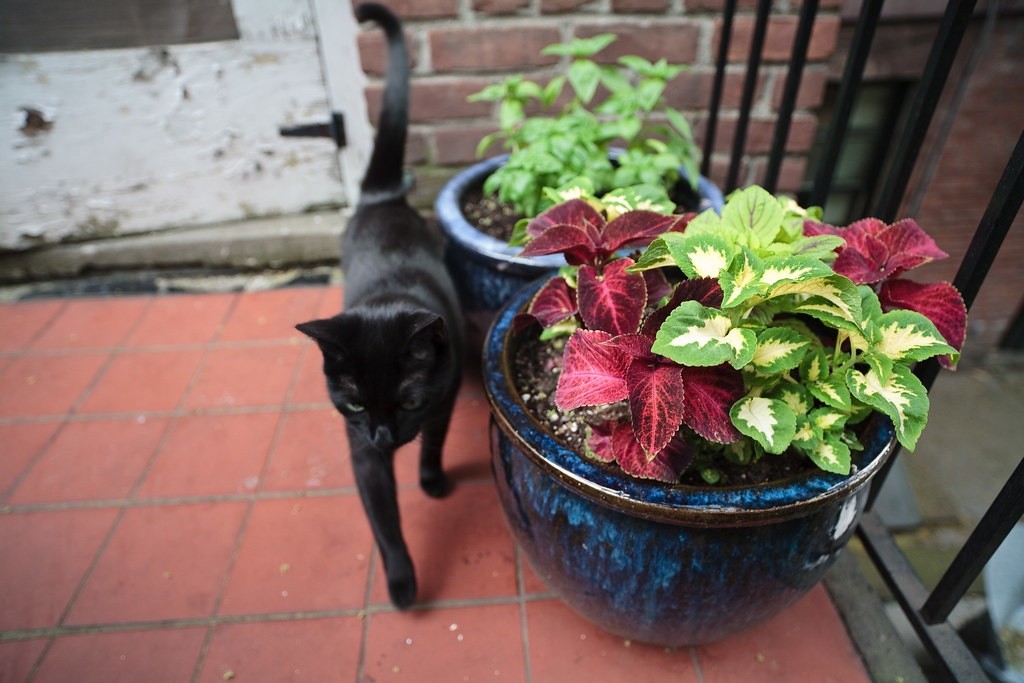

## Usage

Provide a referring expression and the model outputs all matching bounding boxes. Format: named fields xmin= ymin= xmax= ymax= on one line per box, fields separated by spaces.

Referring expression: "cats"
xmin=293 ymin=2 xmax=466 ymax=609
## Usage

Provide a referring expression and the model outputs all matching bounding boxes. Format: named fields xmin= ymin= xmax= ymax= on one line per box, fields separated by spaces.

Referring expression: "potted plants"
xmin=433 ymin=33 xmax=728 ymax=366
xmin=480 ymin=184 xmax=969 ymax=645
